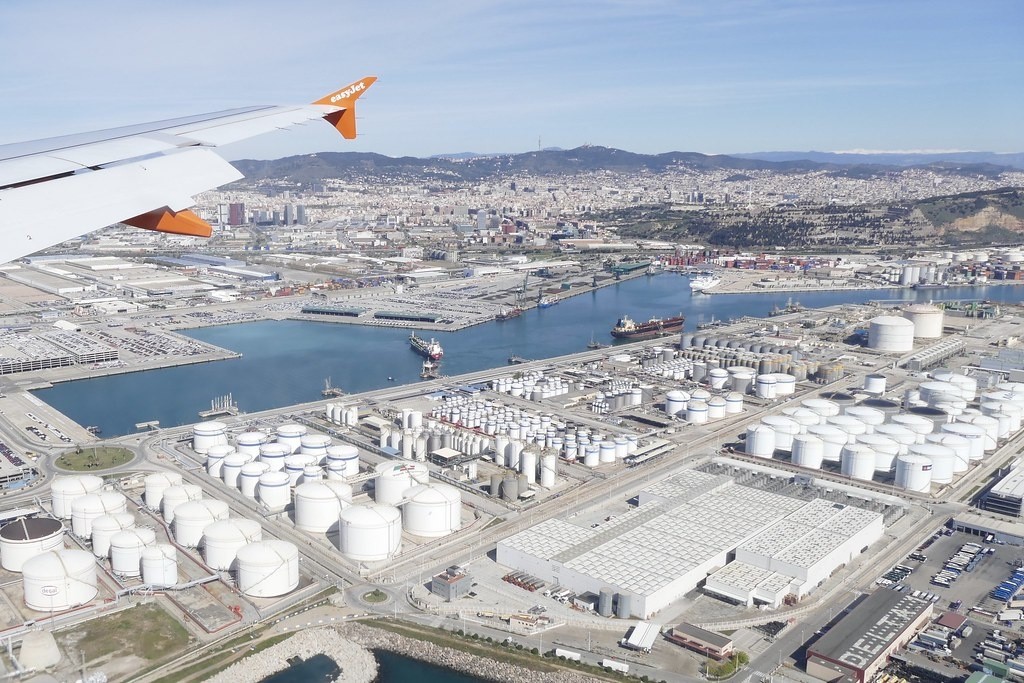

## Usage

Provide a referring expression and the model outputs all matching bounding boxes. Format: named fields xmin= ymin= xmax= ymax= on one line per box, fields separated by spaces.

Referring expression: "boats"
xmin=495 ymin=305 xmax=523 ymax=320
xmin=646 ymin=266 xmax=655 ymax=275
xmin=689 ymin=273 xmax=721 ymax=292
xmin=538 ymin=294 xmax=560 ymax=308
xmin=409 ymin=331 xmax=443 ymax=360
xmin=611 ymin=312 xmax=685 ymax=338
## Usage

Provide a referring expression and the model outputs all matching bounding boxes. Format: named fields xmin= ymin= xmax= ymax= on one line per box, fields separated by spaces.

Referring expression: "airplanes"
xmin=0 ymin=76 xmax=378 ymax=264
